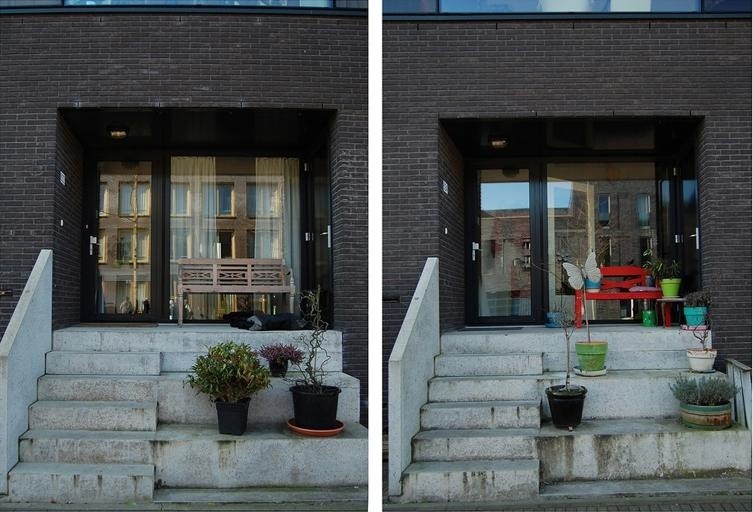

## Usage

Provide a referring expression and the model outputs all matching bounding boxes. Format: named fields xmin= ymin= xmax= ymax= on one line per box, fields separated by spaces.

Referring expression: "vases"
xmin=645 ymin=275 xmax=652 ymax=287
xmin=544 ymin=311 xmax=568 ymax=328
xmin=571 ymin=340 xmax=608 ymax=376
xmin=583 ymin=278 xmax=601 ymax=293
xmin=544 ymin=384 xmax=587 ymax=429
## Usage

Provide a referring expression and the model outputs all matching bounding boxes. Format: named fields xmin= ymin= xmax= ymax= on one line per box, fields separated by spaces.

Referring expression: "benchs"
xmin=177 ymin=258 xmax=296 ymax=327
xmin=575 ymin=264 xmax=670 ymax=328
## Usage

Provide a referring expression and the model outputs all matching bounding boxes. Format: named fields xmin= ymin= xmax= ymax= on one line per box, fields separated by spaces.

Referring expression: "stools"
xmin=654 ymin=298 xmax=686 ymax=327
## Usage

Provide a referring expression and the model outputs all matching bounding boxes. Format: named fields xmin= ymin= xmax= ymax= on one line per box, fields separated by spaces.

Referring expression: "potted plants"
xmin=667 ymin=370 xmax=743 ymax=431
xmin=180 ymin=283 xmax=345 ymax=437
xmin=679 ymin=289 xmax=717 ymax=373
xmin=641 ymin=248 xmax=685 ymax=297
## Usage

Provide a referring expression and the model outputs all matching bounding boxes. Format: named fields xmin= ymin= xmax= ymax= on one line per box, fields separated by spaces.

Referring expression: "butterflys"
xmin=562 ymin=251 xmax=601 ymax=291
